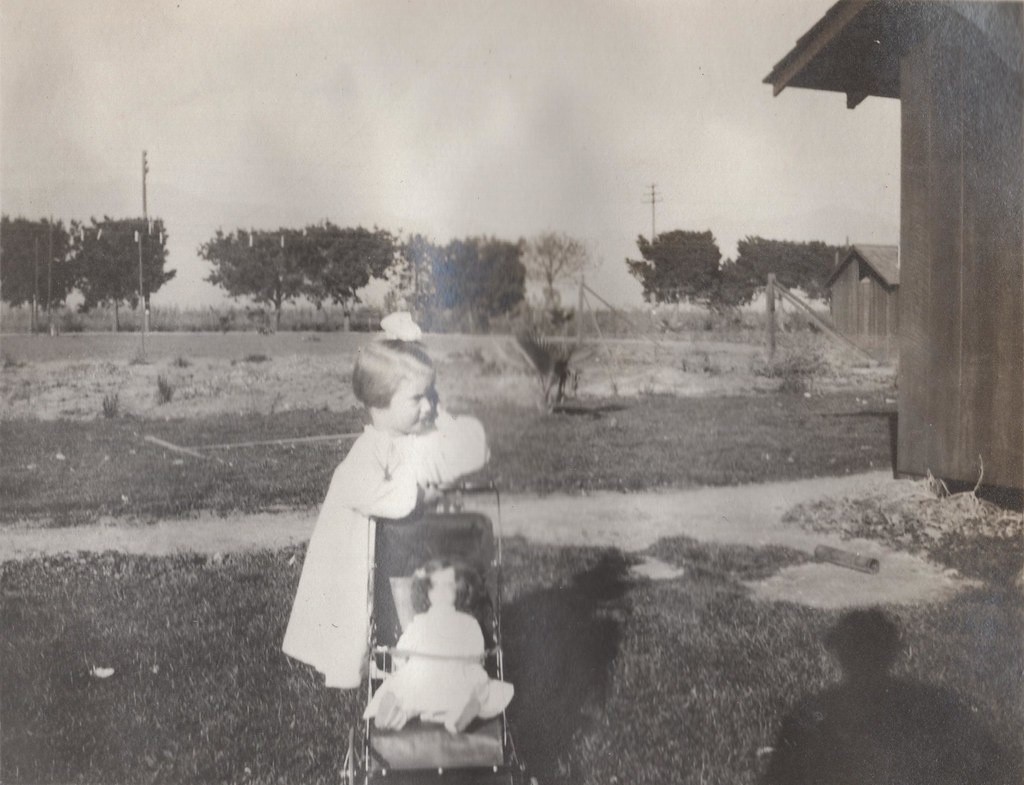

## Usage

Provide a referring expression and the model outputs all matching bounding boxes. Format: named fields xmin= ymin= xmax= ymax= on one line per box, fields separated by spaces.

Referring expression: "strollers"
xmin=343 ymin=480 xmax=526 ymax=784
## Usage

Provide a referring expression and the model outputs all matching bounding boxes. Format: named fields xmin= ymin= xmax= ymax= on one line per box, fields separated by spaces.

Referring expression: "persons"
xmin=279 ymin=339 xmax=492 ymax=689
xmin=363 ymin=558 xmax=513 ymax=735
